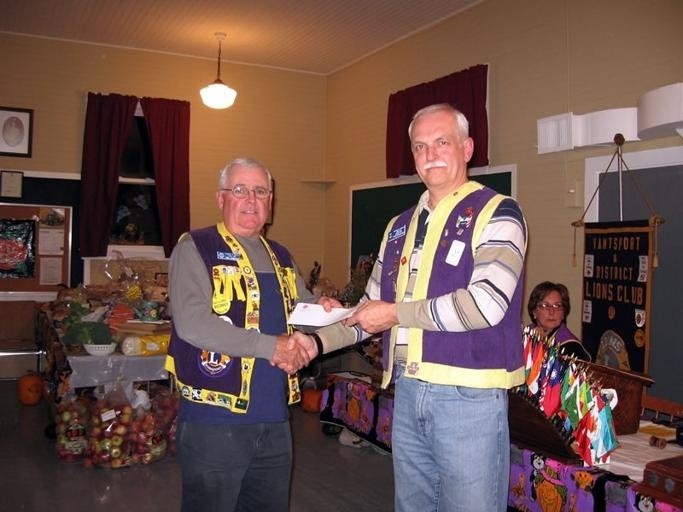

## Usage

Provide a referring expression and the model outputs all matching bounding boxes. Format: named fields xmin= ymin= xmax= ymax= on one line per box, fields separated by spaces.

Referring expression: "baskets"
xmin=83 ymin=342 xmax=118 ymax=356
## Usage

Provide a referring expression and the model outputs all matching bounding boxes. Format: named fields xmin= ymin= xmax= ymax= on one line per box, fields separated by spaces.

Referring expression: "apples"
xmin=55 ymin=382 xmax=180 ymax=469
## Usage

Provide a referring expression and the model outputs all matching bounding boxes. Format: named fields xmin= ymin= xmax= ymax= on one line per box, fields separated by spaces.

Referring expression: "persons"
xmin=157 ymin=157 xmax=351 ymax=511
xmin=518 ymin=278 xmax=590 ymax=361
xmin=265 ymin=103 xmax=530 ymax=511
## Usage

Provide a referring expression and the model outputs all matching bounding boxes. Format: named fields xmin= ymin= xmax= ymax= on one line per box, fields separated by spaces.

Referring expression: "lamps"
xmin=200 ymin=32 xmax=237 ymax=110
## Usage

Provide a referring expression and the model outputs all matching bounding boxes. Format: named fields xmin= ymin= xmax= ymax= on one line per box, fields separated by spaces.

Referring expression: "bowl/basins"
xmin=83 ymin=343 xmax=117 ymax=357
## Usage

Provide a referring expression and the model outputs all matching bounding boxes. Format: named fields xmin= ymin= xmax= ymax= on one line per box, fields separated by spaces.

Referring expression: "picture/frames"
xmin=0 ymin=106 xmax=34 ymax=158
xmin=0 ymin=170 xmax=24 ymax=203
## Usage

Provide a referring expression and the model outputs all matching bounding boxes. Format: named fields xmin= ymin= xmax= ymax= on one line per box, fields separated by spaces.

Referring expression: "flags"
xmin=578 ymin=218 xmax=658 ymax=408
xmin=514 ymin=324 xmax=621 ymax=468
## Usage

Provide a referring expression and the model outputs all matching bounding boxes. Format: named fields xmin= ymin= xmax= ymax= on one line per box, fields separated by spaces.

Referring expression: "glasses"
xmin=537 ymin=302 xmax=564 ymax=311
xmin=221 ymin=183 xmax=272 ymax=200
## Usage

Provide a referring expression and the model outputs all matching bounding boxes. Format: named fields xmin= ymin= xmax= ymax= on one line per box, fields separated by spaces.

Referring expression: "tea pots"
xmin=128 ymin=301 xmax=167 ymax=320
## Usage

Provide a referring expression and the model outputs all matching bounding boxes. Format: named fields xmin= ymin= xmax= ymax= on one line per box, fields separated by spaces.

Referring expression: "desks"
xmin=321 ymin=372 xmax=683 ymax=512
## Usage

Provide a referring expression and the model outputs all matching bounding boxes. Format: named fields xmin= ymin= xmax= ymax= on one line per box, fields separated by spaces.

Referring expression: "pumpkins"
xmin=18 ymin=369 xmax=43 ymax=406
xmin=575 ymin=471 xmax=593 ymax=489
xmin=347 ymin=398 xmax=360 ymax=421
xmin=301 ymin=383 xmax=321 ymax=413
xmin=536 ymin=480 xmax=563 ymax=512
